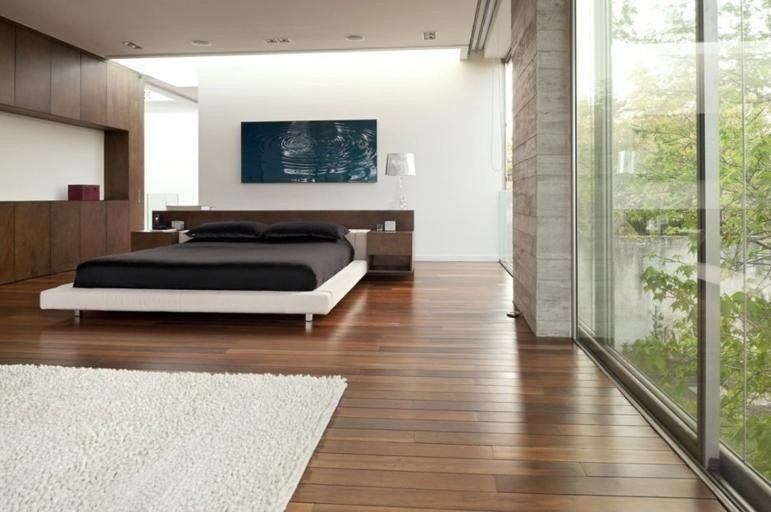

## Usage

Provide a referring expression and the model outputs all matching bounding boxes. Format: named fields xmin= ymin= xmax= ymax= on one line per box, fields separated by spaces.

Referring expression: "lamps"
xmin=384 ymin=152 xmax=416 ymax=211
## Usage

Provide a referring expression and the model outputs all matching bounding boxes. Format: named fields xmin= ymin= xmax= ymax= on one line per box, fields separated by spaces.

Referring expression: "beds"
xmin=39 ymin=210 xmax=414 ymax=322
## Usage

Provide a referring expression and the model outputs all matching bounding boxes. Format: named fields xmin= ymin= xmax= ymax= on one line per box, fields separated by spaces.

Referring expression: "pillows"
xmin=184 ymin=219 xmax=269 ymax=243
xmin=258 ymin=220 xmax=347 ymax=243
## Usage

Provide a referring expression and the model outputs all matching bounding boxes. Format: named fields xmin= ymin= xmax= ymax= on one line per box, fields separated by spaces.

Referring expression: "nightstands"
xmin=366 ymin=230 xmax=415 ymax=281
xmin=130 ymin=229 xmax=179 ymax=252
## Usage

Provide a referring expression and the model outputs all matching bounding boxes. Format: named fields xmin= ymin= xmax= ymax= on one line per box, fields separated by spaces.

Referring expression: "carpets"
xmin=0 ymin=362 xmax=349 ymax=512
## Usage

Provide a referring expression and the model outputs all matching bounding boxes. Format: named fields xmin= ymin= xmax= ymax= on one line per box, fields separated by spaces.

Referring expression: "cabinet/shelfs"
xmin=0 ymin=16 xmax=145 ymax=286
